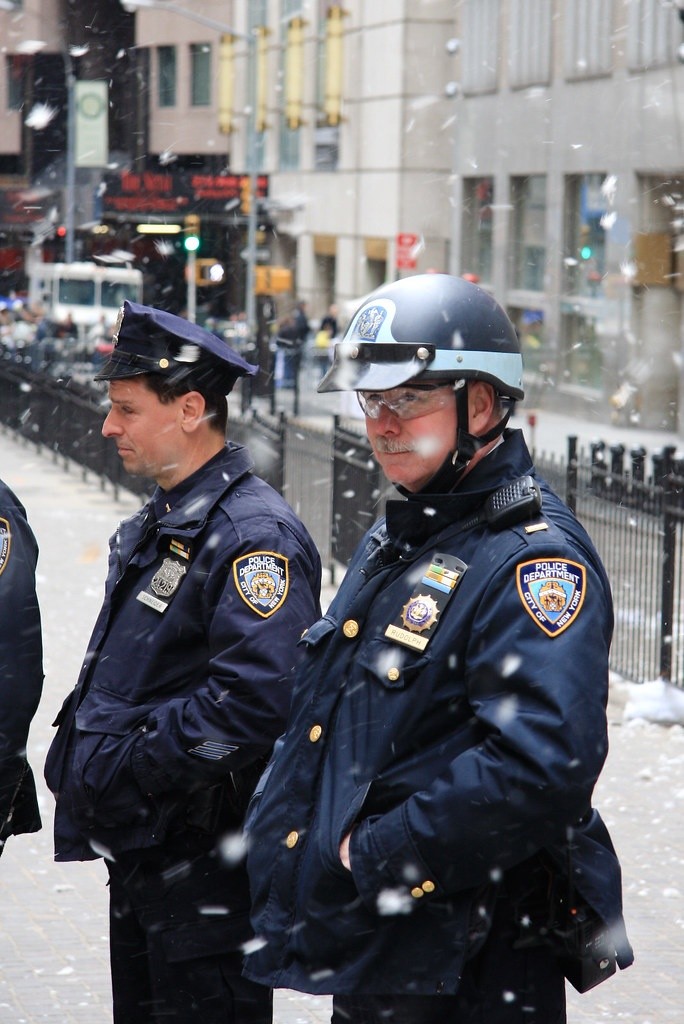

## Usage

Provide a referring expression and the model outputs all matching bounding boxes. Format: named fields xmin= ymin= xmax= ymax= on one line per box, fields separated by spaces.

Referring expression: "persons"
xmin=44 ymin=296 xmax=328 ymax=1024
xmin=273 ymin=300 xmax=340 ymax=389
xmin=236 ymin=272 xmax=640 ymax=1024
xmin=0 ymin=474 xmax=46 ymax=876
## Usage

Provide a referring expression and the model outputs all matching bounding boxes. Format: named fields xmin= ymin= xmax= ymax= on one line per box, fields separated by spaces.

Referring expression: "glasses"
xmin=357 ymin=383 xmax=456 ymax=419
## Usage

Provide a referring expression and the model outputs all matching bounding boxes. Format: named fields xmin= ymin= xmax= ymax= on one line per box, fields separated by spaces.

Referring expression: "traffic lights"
xmin=56 ymin=226 xmax=66 ymax=250
xmin=183 ymin=215 xmax=199 ymax=249
xmin=186 ymin=258 xmax=224 ymax=286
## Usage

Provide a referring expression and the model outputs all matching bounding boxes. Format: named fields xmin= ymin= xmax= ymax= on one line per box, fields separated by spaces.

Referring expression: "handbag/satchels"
xmin=545 ymin=807 xmax=634 ymax=993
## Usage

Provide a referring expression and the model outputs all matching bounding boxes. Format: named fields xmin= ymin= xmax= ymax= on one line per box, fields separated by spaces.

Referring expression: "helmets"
xmin=317 ymin=273 xmax=524 ymax=400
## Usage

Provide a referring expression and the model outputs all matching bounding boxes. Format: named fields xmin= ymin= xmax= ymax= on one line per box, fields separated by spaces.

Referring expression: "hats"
xmin=93 ymin=300 xmax=260 ymax=394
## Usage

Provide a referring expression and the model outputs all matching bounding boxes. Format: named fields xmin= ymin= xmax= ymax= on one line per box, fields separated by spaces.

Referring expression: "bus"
xmin=36 ymin=263 xmax=144 ymax=345
